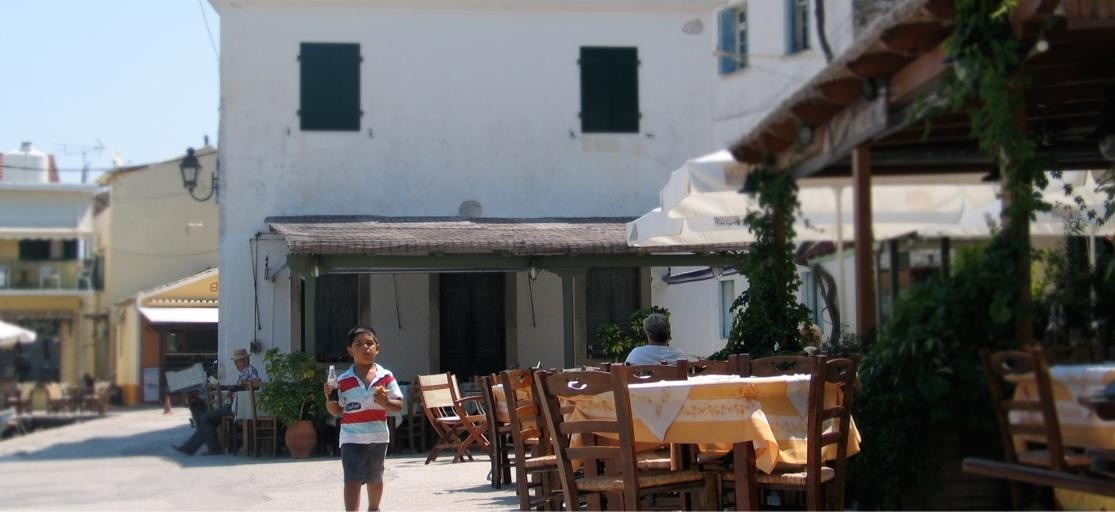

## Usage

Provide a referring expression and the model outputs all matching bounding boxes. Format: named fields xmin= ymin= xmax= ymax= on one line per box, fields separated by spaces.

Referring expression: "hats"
xmin=229 ymin=348 xmax=252 ymax=360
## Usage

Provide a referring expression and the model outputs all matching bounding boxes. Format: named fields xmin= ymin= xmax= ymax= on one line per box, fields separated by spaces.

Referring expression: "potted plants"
xmin=254 ymin=347 xmax=335 ymax=459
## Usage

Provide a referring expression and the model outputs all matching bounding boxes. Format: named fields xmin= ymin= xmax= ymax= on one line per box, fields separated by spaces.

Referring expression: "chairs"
xmin=8 ymin=380 xmax=109 ymax=419
xmin=978 ymin=344 xmax=1096 ymax=509
xmin=39 ymin=259 xmax=95 ymax=289
xmin=205 ymin=382 xmax=279 ymax=459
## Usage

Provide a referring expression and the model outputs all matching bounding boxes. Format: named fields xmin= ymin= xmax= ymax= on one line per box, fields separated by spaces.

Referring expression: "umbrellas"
xmin=622 ymin=202 xmax=961 ymax=246
xmin=0 ymin=318 xmax=40 ymax=351
xmin=655 ymin=142 xmax=1115 ymax=350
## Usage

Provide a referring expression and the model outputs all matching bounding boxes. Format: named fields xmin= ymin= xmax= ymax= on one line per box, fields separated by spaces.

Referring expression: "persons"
xmin=620 ymin=313 xmax=692 ymax=377
xmin=170 ymin=348 xmax=262 ymax=457
xmin=323 ymin=325 xmax=405 ymax=512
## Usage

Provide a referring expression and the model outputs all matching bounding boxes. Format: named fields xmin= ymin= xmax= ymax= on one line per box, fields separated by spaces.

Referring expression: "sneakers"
xmin=200 ymin=451 xmax=225 ymax=455
xmin=170 ymin=443 xmax=191 ymax=456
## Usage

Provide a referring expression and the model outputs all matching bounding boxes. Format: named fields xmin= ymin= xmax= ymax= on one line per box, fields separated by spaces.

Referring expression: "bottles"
xmin=326 ymin=365 xmax=339 ymax=405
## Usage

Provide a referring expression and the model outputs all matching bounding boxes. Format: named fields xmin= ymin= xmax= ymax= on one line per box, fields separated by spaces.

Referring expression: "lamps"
xmin=179 ymin=147 xmax=219 ymax=203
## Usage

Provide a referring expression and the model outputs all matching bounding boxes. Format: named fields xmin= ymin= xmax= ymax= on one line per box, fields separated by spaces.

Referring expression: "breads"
xmin=375 ymin=386 xmax=389 ymax=396
